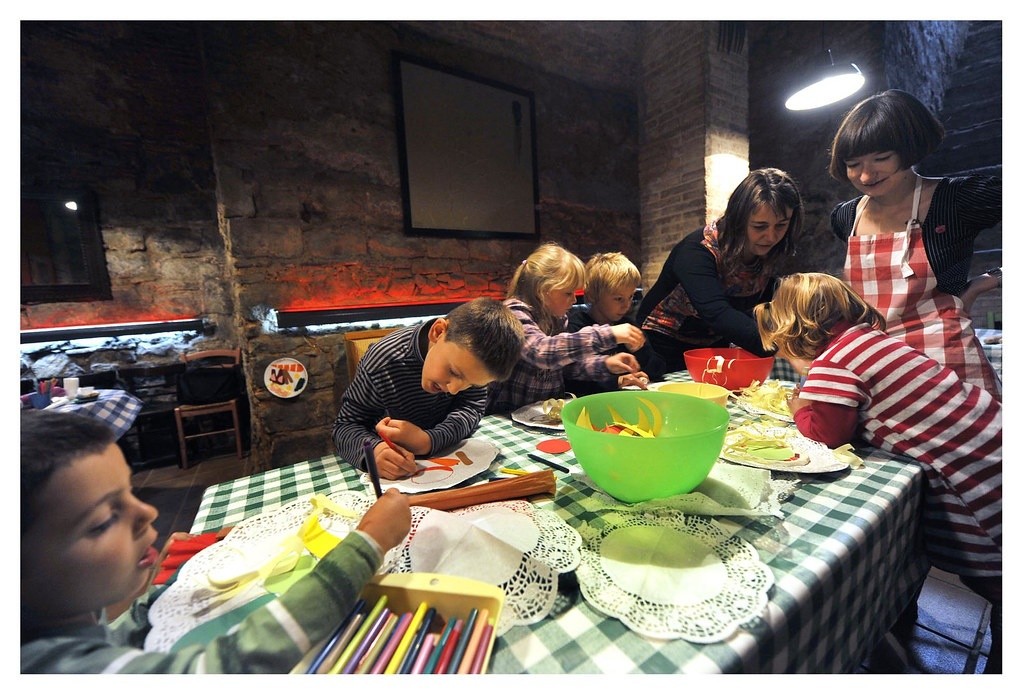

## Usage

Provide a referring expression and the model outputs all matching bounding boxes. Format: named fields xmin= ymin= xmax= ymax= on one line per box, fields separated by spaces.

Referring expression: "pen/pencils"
xmin=379 ymin=431 xmax=405 ymax=459
xmin=308 ymin=595 xmax=494 ymax=675
xmin=526 ymin=454 xmax=570 ymax=473
xmin=32 ymin=377 xmax=59 ymax=394
xmin=500 ymin=468 xmax=557 ymax=481
xmin=364 ymin=441 xmax=383 ymax=500
xmin=489 ymin=477 xmax=506 ymax=481
xmin=799 ymin=367 xmax=809 ymax=389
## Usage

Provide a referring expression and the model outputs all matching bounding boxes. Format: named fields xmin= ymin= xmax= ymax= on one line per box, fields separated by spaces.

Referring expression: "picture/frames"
xmin=391 ymin=45 xmax=543 ymax=242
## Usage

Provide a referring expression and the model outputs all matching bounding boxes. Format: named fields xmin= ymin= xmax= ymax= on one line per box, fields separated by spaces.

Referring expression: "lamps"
xmin=781 ymin=21 xmax=866 ymax=111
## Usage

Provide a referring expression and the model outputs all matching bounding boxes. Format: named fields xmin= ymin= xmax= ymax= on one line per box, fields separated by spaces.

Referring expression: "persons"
xmin=331 ymin=298 xmax=524 ymax=480
xmin=830 ymin=89 xmax=1002 ymax=400
xmin=755 ymin=277 xmax=1002 ymax=598
xmin=568 ymin=252 xmax=662 ymax=391
xmin=20 ymin=408 xmax=412 ymax=674
xmin=632 ymin=171 xmax=805 ymax=379
xmin=485 ymin=244 xmax=645 ymax=416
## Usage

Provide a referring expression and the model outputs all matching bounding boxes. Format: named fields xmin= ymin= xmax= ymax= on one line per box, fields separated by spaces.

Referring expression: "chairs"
xmin=173 ymin=345 xmax=245 ymax=467
xmin=344 ymin=328 xmax=401 ymax=384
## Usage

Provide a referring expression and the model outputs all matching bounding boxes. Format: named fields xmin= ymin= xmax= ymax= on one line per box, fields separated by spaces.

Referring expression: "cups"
xmin=63 ymin=378 xmax=79 ymax=399
xmin=30 ymin=392 xmax=52 ymax=409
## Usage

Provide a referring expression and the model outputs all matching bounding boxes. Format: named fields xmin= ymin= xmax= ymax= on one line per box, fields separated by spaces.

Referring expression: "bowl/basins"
xmin=559 ymin=391 xmax=730 ymax=503
xmin=658 ymin=382 xmax=728 ymax=406
xmin=684 ymin=347 xmax=775 ymax=395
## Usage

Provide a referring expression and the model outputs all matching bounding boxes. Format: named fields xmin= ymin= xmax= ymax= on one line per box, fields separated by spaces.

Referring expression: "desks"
xmin=190 ymin=373 xmax=924 ymax=674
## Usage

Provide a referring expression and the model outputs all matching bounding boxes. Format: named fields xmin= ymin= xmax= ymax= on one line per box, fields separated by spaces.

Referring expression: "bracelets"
xmin=986 ymin=268 xmax=1002 ymax=288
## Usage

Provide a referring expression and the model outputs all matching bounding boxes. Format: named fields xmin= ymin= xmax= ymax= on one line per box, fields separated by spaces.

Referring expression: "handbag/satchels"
xmin=176 ymin=360 xmax=241 ymax=405
